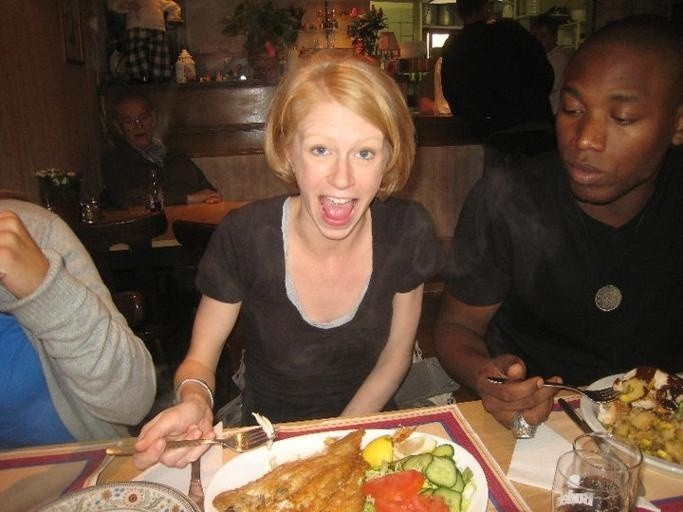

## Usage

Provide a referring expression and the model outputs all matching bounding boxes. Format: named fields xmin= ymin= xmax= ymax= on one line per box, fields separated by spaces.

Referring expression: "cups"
xmin=551 ymin=449 xmax=631 ymax=512
xmin=572 ymin=433 xmax=643 ymax=511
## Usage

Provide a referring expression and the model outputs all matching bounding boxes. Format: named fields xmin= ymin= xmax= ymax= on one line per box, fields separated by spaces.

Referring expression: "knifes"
xmin=557 ymin=396 xmax=647 ymax=497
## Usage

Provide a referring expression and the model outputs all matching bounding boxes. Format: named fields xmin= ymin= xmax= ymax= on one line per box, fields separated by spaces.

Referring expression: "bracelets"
xmin=174 ymin=377 xmax=215 ymax=409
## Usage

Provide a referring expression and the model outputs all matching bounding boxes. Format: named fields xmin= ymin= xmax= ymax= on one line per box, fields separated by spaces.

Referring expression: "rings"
xmin=509 ymin=409 xmax=536 ymax=439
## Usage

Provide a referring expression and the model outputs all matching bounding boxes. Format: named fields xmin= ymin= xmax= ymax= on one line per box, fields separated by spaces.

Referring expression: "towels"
xmin=505 ymin=418 xmax=663 ymax=511
xmin=120 ymin=420 xmax=224 ymax=503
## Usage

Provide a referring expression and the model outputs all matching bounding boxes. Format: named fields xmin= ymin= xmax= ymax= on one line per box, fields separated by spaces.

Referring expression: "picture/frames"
xmin=55 ymin=0 xmax=87 ymax=68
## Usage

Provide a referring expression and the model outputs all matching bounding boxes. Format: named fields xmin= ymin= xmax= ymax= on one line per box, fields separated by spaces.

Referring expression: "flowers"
xmin=344 ymin=4 xmax=387 ymax=55
xmin=34 ymin=167 xmax=83 ymax=206
xmin=219 ymin=1 xmax=304 ymax=54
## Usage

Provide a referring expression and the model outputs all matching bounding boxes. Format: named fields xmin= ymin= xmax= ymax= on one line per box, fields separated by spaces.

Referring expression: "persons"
xmin=439 ymin=1 xmax=562 ymax=180
xmin=99 ymin=93 xmax=221 ymax=206
xmin=105 ymin=0 xmax=182 ymax=81
xmin=432 ymin=17 xmax=682 ymax=439
xmin=132 ymin=47 xmax=444 ymax=470
xmin=0 ymin=197 xmax=158 ymax=452
xmin=526 ymin=13 xmax=572 ymax=116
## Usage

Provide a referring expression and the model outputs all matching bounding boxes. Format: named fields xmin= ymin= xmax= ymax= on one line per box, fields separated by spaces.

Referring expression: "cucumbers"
xmin=394 ymin=444 xmax=465 ymax=512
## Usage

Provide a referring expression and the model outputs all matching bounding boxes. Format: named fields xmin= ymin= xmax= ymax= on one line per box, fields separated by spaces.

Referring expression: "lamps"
xmin=375 ymin=31 xmax=401 ymax=56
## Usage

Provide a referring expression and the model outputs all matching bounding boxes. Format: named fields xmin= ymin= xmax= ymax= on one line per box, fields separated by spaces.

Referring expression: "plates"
xmin=203 ymin=427 xmax=488 ymax=512
xmin=24 ymin=479 xmax=200 ymax=511
xmin=580 ymin=372 xmax=682 ymax=476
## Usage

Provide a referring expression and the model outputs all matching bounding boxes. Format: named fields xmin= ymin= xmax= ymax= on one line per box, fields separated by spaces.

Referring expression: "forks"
xmin=485 ymin=375 xmax=619 ymax=404
xmin=104 ymin=423 xmax=281 ymax=456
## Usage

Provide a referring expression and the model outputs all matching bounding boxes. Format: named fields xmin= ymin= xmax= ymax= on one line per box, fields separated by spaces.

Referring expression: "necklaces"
xmin=559 ymin=179 xmax=658 ymax=313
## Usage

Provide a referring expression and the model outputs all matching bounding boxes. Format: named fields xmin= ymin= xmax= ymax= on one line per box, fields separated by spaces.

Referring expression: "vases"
xmin=364 ymin=56 xmax=381 ymax=68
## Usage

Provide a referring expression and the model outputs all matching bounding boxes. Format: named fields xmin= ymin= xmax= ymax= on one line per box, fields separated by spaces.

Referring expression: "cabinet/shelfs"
xmin=410 ymin=0 xmax=582 ymax=60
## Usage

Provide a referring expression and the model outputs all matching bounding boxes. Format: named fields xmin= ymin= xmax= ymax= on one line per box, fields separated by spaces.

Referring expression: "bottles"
xmin=175 ymin=49 xmax=198 ymax=82
xmin=79 ymin=198 xmax=103 ymax=223
xmin=143 ymin=165 xmax=163 ymax=212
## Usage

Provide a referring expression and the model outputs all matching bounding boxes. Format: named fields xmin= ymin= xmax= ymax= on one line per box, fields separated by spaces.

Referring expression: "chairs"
xmin=177 ymin=218 xmax=247 ymax=397
xmin=76 ymin=210 xmax=171 ymax=375
